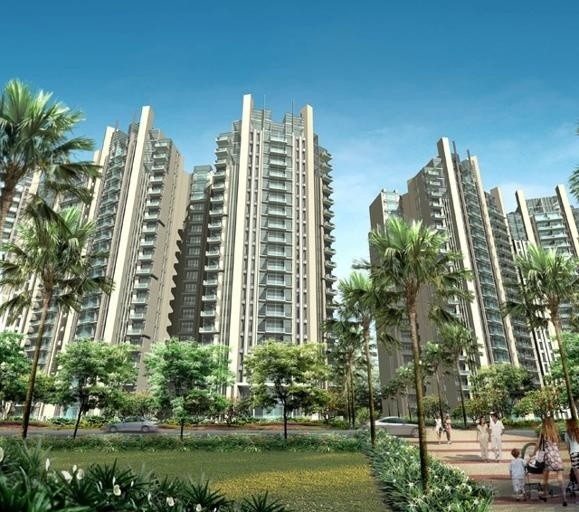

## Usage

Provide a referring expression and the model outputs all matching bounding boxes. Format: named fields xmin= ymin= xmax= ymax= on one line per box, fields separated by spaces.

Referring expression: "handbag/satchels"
xmin=536 ymin=449 xmax=547 ymax=466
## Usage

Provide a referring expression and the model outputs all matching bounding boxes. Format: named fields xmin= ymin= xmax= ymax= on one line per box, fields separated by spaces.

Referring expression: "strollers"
xmin=566 ymin=468 xmax=579 ymax=498
xmin=521 ymin=442 xmax=555 ymax=501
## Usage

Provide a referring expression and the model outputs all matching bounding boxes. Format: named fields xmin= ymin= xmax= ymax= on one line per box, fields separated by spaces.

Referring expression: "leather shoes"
xmin=538 ymin=494 xmax=547 ymax=502
xmin=562 ymin=501 xmax=568 ymax=507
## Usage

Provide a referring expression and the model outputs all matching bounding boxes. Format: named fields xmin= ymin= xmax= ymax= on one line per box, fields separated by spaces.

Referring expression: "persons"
xmin=434 ymin=413 xmax=444 ymax=444
xmin=476 ymin=416 xmax=490 ymax=462
xmin=563 ymin=419 xmax=579 ymax=484
xmin=509 ymin=449 xmax=527 ymax=501
xmin=529 ymin=417 xmax=568 ymax=507
xmin=488 ymin=413 xmax=505 ymax=462
xmin=444 ymin=414 xmax=452 ymax=444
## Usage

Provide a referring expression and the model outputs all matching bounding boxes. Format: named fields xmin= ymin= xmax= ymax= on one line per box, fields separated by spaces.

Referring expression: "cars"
xmin=367 ymin=415 xmax=419 ymax=437
xmin=102 ymin=415 xmax=159 ymax=433
